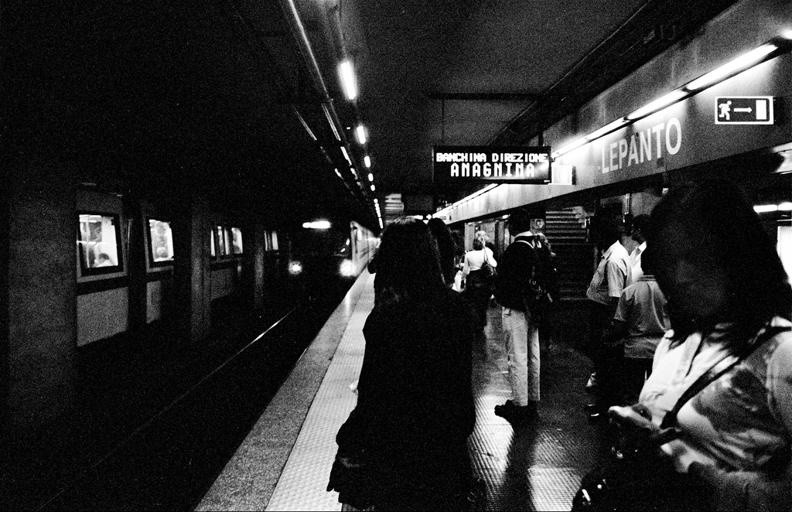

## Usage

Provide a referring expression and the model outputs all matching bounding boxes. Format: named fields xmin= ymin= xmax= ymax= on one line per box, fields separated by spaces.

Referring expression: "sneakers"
xmin=494 ymin=398 xmax=539 ymax=417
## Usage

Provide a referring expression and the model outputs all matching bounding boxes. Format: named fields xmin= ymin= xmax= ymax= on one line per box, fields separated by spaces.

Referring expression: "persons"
xmin=326 ymin=178 xmax=791 ymax=511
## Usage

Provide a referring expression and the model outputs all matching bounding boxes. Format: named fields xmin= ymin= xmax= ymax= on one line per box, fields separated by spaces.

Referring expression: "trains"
xmin=276 ymin=212 xmax=383 ymax=284
xmin=0 ymin=118 xmax=286 ymax=461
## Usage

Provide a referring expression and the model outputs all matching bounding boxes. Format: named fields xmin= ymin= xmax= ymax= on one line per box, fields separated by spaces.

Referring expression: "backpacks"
xmin=517 ymin=239 xmax=561 ymax=315
